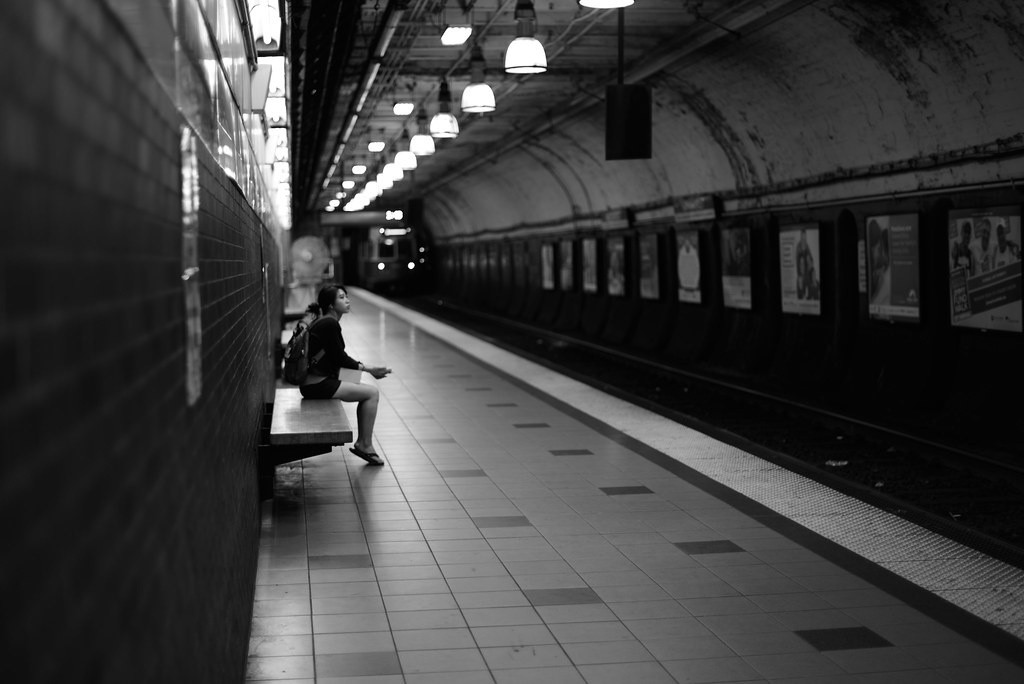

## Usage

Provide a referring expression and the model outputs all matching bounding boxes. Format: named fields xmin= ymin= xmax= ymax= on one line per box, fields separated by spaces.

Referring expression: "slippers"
xmin=349 ymin=446 xmax=385 ymax=465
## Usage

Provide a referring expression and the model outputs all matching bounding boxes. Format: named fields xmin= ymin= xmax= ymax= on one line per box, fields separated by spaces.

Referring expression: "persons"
xmin=949 ymin=219 xmax=1022 ymax=276
xmin=299 ymin=285 xmax=392 ymax=466
xmin=794 ymin=231 xmax=824 ymax=303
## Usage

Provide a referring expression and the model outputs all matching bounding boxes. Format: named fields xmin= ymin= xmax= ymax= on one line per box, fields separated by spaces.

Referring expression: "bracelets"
xmin=361 ymin=364 xmax=365 ymax=372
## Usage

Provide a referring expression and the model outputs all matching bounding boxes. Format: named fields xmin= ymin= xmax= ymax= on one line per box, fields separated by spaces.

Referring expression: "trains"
xmin=331 ymin=224 xmax=431 ymax=298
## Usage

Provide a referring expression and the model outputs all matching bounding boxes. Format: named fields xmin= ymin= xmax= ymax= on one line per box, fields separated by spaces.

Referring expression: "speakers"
xmin=604 ymin=83 xmax=654 ymax=161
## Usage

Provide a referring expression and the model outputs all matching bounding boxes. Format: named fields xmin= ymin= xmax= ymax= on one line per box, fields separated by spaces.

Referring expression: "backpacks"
xmin=282 ymin=313 xmax=338 ymax=386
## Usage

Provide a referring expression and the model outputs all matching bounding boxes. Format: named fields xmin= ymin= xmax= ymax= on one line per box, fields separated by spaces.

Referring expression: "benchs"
xmin=256 ymin=379 xmax=353 ymax=501
xmin=280 ymin=329 xmax=293 ymax=364
xmin=284 ymin=305 xmax=306 ymax=329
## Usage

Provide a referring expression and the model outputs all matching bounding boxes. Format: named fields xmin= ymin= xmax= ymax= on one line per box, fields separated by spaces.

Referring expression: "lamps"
xmin=394 ymin=128 xmax=418 ymax=171
xmin=429 ymin=75 xmax=460 ymax=139
xmin=438 ymin=0 xmax=475 ymax=47
xmin=503 ymin=0 xmax=548 ymax=76
xmin=255 ymin=54 xmax=293 ymax=184
xmin=365 ymin=143 xmax=404 ymax=197
xmin=460 ymin=44 xmax=497 ymax=113
xmin=409 ymin=105 xmax=436 ymax=157
xmin=242 ymin=0 xmax=288 ymax=58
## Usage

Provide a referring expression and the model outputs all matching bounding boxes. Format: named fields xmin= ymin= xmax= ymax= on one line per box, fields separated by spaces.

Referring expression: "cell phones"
xmin=379 ymin=369 xmax=392 ymax=374
